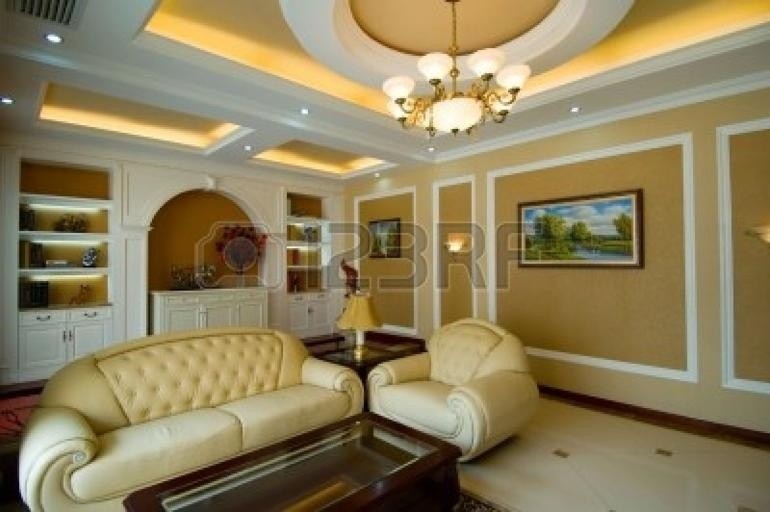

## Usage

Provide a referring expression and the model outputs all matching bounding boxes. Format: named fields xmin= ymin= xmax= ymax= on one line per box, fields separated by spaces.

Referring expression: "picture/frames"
xmin=518 ymin=187 xmax=646 ymax=269
xmin=368 ymin=217 xmax=402 ymax=259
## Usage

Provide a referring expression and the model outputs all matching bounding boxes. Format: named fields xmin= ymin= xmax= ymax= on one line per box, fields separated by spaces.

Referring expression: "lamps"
xmin=335 ymin=294 xmax=384 ymax=356
xmin=382 ymin=1 xmax=532 ymax=138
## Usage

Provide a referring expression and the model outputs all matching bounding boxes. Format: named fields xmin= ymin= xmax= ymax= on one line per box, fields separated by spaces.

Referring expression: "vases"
xmin=236 ymin=271 xmax=245 ymax=291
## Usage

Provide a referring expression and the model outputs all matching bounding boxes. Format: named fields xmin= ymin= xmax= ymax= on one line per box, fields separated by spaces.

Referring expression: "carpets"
xmin=1 ymin=431 xmax=515 ymax=512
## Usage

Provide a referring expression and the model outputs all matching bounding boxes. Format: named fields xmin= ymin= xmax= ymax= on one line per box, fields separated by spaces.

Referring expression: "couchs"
xmin=17 ymin=325 xmax=365 ymax=512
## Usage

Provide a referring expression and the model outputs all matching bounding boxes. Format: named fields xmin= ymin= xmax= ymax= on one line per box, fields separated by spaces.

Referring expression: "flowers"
xmin=216 ymin=224 xmax=266 ymax=272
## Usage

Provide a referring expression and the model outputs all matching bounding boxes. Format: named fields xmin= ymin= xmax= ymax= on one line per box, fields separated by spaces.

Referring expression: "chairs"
xmin=366 ymin=318 xmax=541 ymax=464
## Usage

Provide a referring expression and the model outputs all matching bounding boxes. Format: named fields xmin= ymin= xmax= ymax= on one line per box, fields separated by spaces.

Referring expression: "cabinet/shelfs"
xmin=14 ymin=149 xmax=114 ymax=376
xmin=300 ymin=330 xmax=426 ymax=378
xmin=149 ymin=285 xmax=269 ymax=335
xmin=283 ymin=190 xmax=330 ymax=339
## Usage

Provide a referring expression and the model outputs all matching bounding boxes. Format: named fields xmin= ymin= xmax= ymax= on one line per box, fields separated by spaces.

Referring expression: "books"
xmin=19 ymin=208 xmax=49 ymax=310
xmin=44 ymin=258 xmax=71 ymax=267
